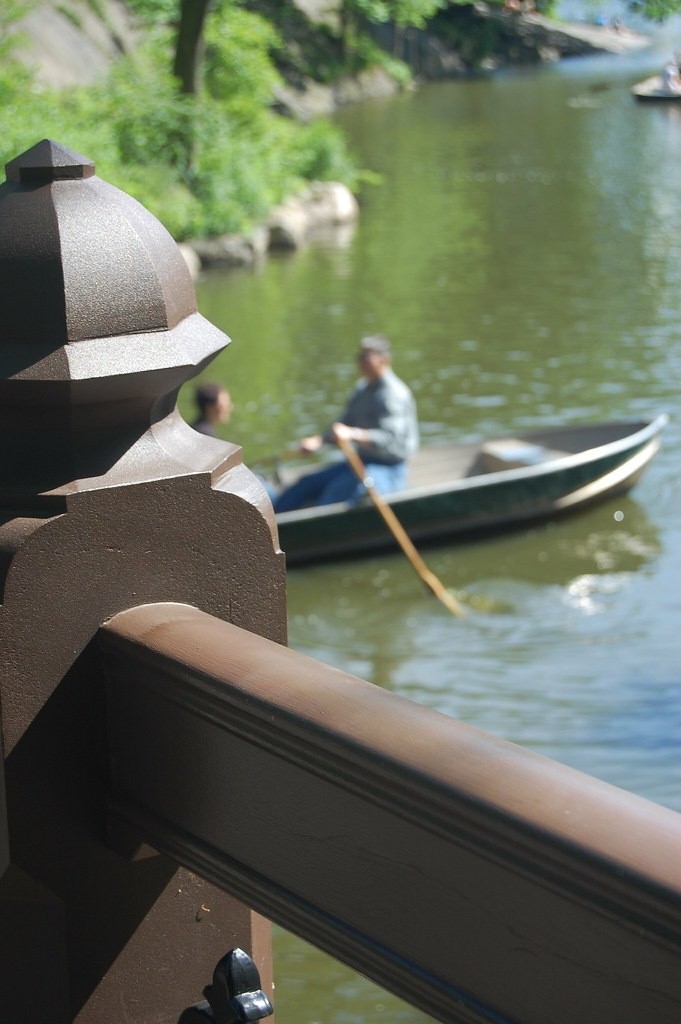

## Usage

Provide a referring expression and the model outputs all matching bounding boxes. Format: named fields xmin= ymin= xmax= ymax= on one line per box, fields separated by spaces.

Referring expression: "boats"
xmin=626 ymin=75 xmax=679 ymax=98
xmin=255 ymin=416 xmax=669 ymax=569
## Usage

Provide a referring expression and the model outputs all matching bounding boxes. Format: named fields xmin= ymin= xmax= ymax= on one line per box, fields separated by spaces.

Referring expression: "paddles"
xmin=246 ymin=449 xmax=310 ymax=470
xmin=331 ymin=420 xmax=464 ymax=618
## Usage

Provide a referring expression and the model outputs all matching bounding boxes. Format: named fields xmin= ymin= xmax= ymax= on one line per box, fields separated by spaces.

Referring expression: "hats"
xmin=357 ymin=336 xmax=389 ymax=355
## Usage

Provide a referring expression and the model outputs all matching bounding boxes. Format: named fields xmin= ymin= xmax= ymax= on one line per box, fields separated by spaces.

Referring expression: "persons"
xmin=277 ymin=336 xmax=419 ymax=512
xmin=188 ymin=379 xmax=234 ymax=437
